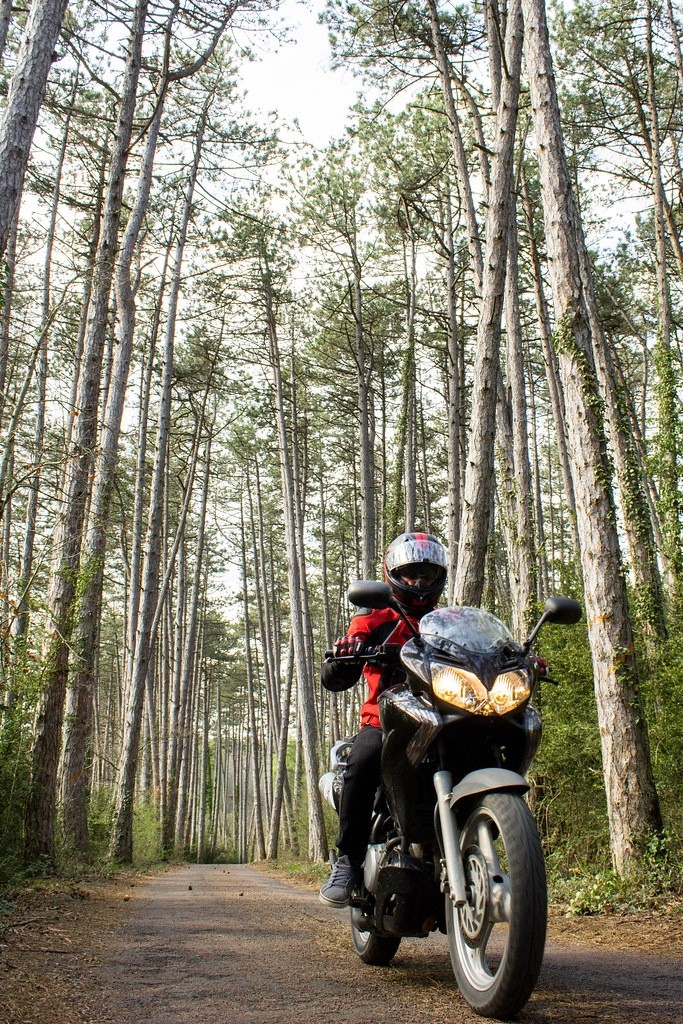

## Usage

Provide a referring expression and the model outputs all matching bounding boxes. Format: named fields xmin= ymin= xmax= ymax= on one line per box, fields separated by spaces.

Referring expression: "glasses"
xmin=397 ymin=565 xmax=438 ymax=581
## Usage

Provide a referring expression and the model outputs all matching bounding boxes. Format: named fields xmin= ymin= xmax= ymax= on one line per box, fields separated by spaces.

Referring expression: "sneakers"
xmin=319 ymin=855 xmax=356 ymax=907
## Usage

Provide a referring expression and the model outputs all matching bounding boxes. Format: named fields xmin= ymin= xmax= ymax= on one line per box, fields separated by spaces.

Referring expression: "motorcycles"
xmin=319 ymin=579 xmax=582 ymax=1018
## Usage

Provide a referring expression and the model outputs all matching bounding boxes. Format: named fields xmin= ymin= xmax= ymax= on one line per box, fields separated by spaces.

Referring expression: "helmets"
xmin=384 ymin=532 xmax=447 ymax=617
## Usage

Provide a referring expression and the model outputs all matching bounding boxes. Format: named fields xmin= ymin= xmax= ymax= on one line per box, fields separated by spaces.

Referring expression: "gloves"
xmin=526 ymin=655 xmax=549 ymax=675
xmin=333 ymin=635 xmax=365 ymax=665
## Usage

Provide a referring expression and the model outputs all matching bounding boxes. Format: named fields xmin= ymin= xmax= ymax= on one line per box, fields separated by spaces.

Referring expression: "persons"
xmin=320 ymin=532 xmax=548 ymax=909
